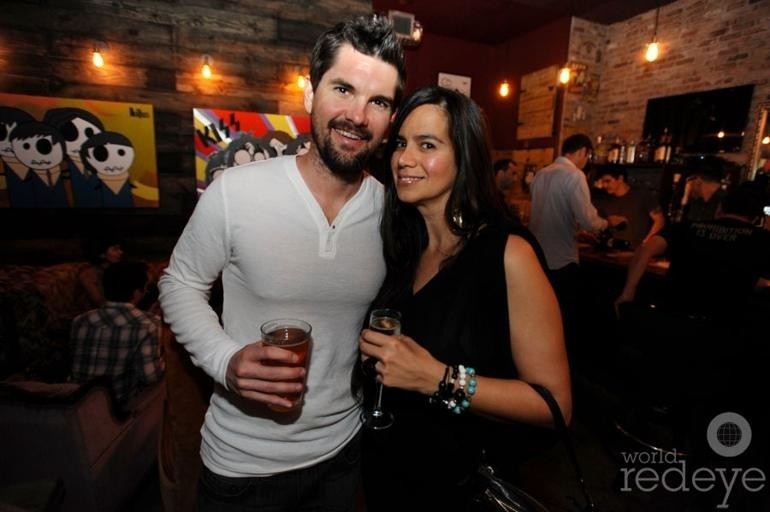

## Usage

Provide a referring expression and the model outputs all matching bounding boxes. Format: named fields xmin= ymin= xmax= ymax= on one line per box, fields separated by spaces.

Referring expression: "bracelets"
xmin=429 ymin=359 xmax=476 ymax=413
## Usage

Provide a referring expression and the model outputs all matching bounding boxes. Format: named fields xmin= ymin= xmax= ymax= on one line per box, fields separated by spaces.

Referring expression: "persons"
xmin=155 ymin=14 xmax=405 ymax=511
xmin=529 ymin=134 xmax=630 ymax=287
xmin=601 ymin=166 xmax=666 ymax=253
xmin=615 ymin=180 xmax=769 ymax=328
xmin=493 ymin=159 xmax=520 ymax=201
xmin=60 ymin=254 xmax=177 ymax=383
xmin=360 ymin=85 xmax=573 ymax=512
xmin=677 ymin=158 xmax=725 ymax=217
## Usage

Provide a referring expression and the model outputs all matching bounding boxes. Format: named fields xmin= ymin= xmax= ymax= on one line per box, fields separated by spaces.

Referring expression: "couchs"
xmin=1 ymin=360 xmax=174 ymax=511
xmin=6 ymin=261 xmax=170 ymax=367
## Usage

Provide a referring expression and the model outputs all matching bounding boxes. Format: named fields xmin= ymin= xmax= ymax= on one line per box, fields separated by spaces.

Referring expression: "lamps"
xmin=646 ymin=34 xmax=660 ymax=61
xmin=295 ymin=64 xmax=307 ymax=89
xmin=89 ymin=42 xmax=104 ymax=67
xmin=198 ymin=57 xmax=213 ymax=80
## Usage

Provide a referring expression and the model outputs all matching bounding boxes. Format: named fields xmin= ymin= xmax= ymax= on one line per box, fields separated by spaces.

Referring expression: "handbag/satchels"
xmin=467 ymin=383 xmax=595 ymax=511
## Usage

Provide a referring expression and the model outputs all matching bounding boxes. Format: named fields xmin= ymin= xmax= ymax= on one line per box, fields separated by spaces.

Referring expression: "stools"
xmin=613 ymin=301 xmax=718 ymax=482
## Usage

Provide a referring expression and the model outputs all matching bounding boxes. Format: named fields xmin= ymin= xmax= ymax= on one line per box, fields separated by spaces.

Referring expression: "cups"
xmin=260 ymin=319 xmax=312 ymax=424
xmin=361 ymin=310 xmax=401 ymax=428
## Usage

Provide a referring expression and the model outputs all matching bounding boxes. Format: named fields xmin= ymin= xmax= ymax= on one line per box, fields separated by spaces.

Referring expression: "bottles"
xmin=607 ymin=135 xmax=624 ymax=165
xmin=654 ymin=127 xmax=673 ymax=164
xmin=623 ymin=140 xmax=636 ymax=164
